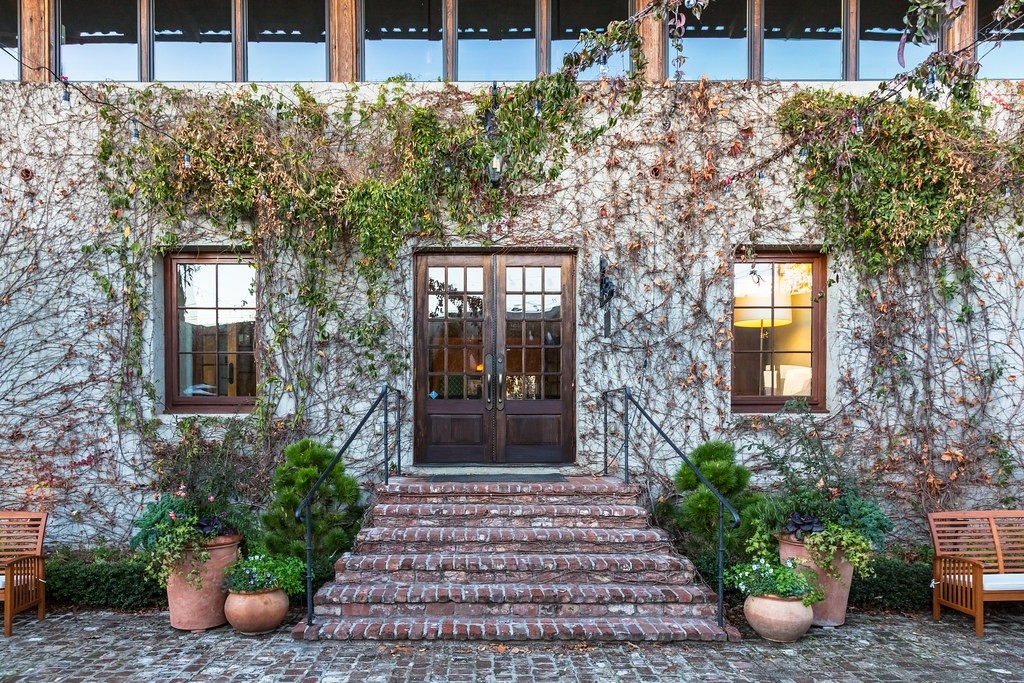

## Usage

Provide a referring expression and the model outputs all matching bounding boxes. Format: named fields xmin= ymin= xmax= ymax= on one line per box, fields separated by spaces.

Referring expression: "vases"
xmin=224 ymin=588 xmax=290 ymax=635
xmin=774 ymin=534 xmax=853 ymax=625
xmin=743 ymin=592 xmax=815 ymax=643
xmin=162 ymin=534 xmax=243 ymax=629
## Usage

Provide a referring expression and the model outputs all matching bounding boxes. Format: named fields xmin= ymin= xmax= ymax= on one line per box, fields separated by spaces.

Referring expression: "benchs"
xmin=0 ymin=509 xmax=48 ymax=637
xmin=927 ymin=509 xmax=1024 ymax=637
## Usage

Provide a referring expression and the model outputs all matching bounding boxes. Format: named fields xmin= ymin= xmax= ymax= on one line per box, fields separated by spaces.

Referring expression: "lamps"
xmin=733 ymin=295 xmax=793 ymax=395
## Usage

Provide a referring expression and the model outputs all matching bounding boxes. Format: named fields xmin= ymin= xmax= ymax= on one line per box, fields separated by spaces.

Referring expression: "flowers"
xmin=145 ymin=477 xmax=279 ymax=593
xmin=225 ymin=552 xmax=308 ymax=595
xmin=722 ymin=531 xmax=825 ymax=608
xmin=737 ymin=396 xmax=894 ymax=587
